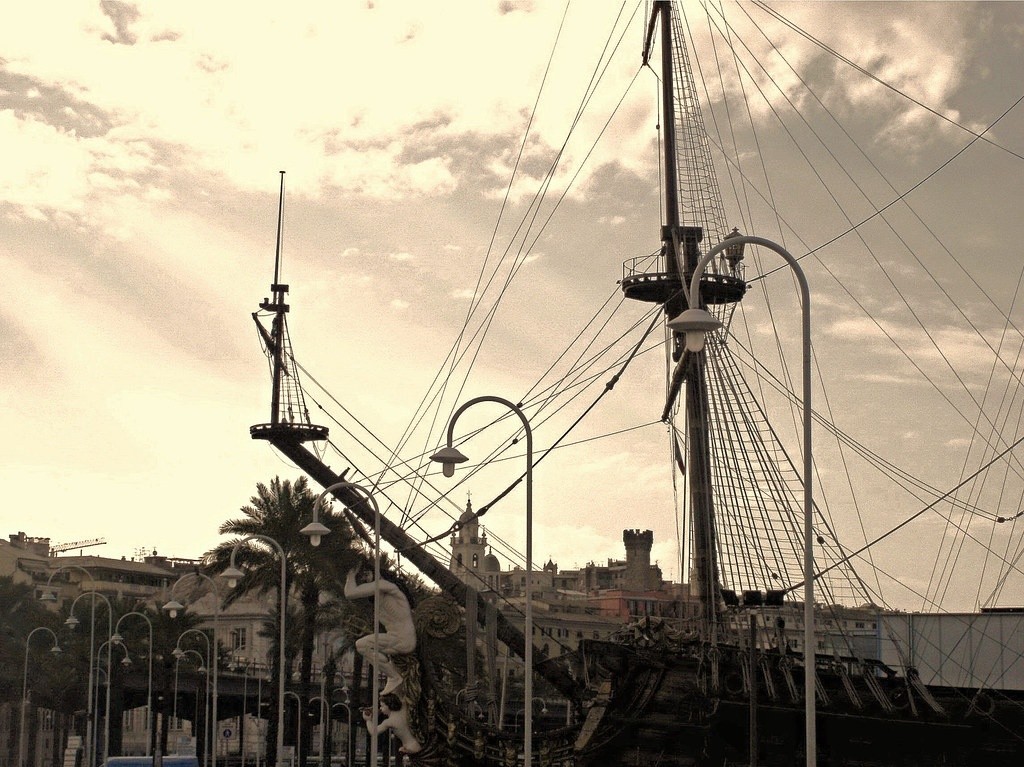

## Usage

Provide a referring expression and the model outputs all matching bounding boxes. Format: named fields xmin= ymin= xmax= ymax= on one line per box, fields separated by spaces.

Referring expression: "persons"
xmin=346 ymin=560 xmax=417 ymax=695
xmin=361 ymin=690 xmax=421 ymax=763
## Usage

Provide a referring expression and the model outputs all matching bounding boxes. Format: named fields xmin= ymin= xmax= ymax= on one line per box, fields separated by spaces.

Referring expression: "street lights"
xmin=668 ymin=237 xmax=816 ymax=767
xmin=18 ymin=482 xmax=380 ymax=767
xmin=429 ymin=395 xmax=533 ymax=767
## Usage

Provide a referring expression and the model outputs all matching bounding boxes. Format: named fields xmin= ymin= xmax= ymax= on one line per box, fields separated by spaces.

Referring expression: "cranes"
xmin=48 ymin=538 xmax=108 ymax=552
